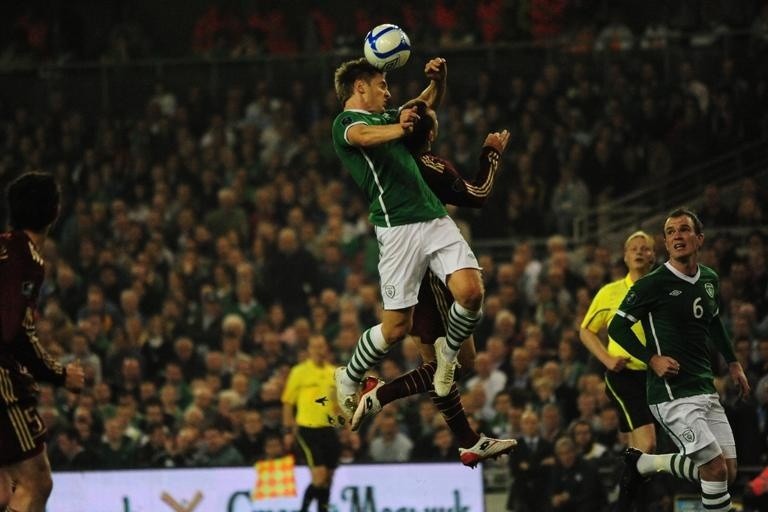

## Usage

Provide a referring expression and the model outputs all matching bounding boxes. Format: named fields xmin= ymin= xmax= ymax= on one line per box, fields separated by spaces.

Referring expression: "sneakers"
xmin=334 ymin=367 xmax=357 ymax=423
xmin=432 ymin=337 xmax=461 ymax=397
xmin=350 ymin=375 xmax=385 ymax=431
xmin=458 ymin=433 xmax=517 ymax=470
xmin=619 ymin=448 xmax=647 ymax=507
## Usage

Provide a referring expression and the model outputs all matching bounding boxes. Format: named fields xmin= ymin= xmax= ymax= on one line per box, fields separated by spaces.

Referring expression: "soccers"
xmin=362 ymin=23 xmax=412 ymax=71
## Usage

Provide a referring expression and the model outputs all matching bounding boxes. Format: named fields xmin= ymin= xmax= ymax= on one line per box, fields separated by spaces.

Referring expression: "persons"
xmin=348 ymin=109 xmax=517 ymax=471
xmin=329 ymin=56 xmax=484 ymax=421
xmin=2 ymin=0 xmax=412 ymax=471
xmin=0 ymin=169 xmax=83 ymax=510
xmin=410 ymin=209 xmax=768 ymax=512
xmin=401 ymin=9 xmax=767 ymax=250
xmin=279 ymin=334 xmax=345 ymax=511
